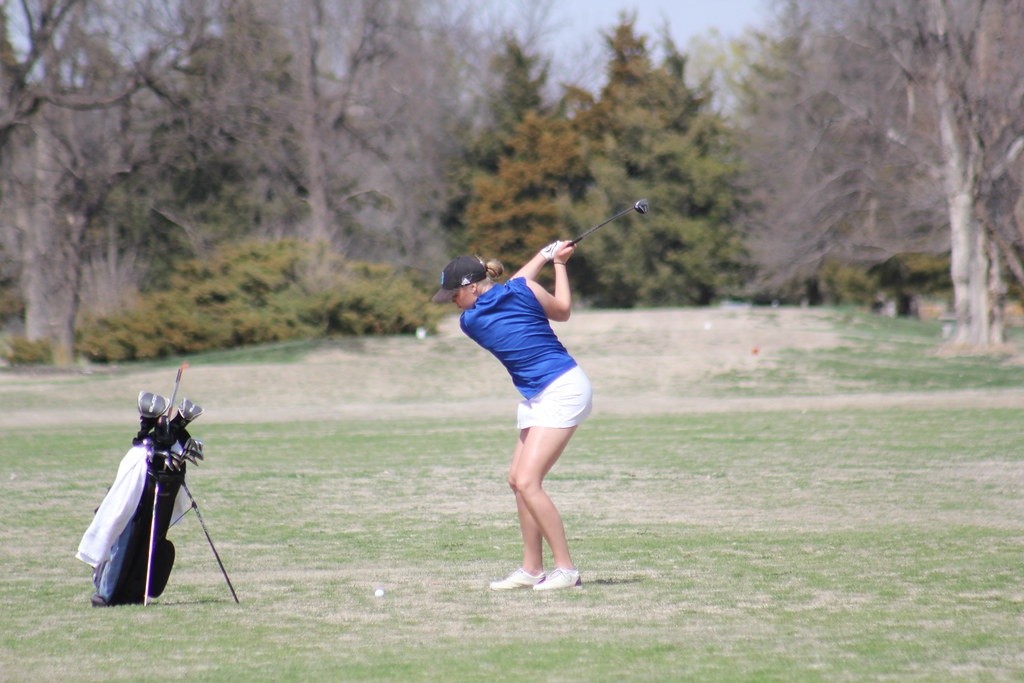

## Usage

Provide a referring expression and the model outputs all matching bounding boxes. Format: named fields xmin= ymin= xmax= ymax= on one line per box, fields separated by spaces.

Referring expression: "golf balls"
xmin=375 ymin=589 xmax=384 ymax=597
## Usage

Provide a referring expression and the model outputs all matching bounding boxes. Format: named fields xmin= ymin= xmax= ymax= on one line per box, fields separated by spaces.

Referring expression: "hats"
xmin=432 ymin=256 xmax=487 ymax=303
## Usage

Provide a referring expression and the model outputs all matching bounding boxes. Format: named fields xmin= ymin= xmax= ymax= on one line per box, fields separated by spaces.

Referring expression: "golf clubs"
xmin=569 ymin=199 xmax=650 ymax=248
xmin=137 ymin=366 xmax=205 ymax=471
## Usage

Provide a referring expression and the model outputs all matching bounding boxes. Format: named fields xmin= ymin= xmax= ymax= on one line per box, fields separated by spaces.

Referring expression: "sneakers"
xmin=490 ymin=568 xmax=544 ymax=591
xmin=534 ymin=569 xmax=582 ymax=593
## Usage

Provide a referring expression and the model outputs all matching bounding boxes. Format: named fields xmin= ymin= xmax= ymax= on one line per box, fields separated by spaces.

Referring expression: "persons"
xmin=429 ymin=239 xmax=593 ymax=592
